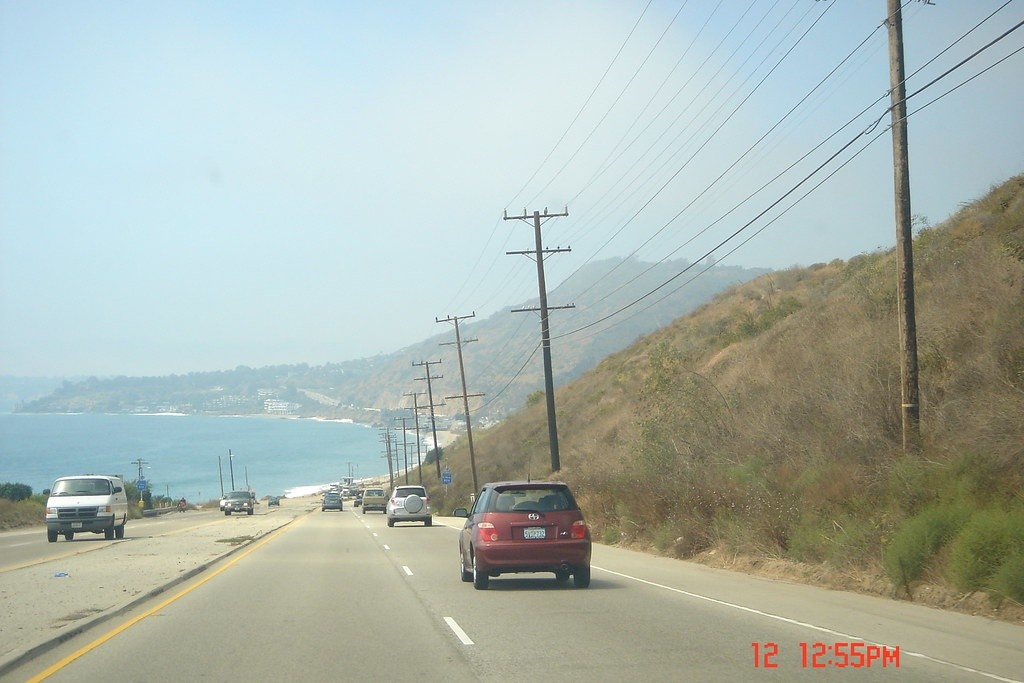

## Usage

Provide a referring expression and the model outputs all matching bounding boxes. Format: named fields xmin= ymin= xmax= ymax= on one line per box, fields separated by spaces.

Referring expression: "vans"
xmin=44 ymin=475 xmax=129 ymax=543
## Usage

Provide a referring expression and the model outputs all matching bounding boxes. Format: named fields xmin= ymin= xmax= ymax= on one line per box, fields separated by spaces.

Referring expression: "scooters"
xmin=179 ymin=505 xmax=187 ymax=513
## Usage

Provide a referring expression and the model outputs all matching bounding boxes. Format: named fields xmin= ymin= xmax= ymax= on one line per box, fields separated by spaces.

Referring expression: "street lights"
xmin=139 ymin=467 xmax=152 ymax=501
xmin=218 ymin=455 xmax=235 ymax=498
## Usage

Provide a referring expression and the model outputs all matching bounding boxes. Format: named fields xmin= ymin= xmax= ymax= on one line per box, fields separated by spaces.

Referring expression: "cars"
xmin=323 ymin=484 xmax=349 ymax=511
xmin=268 ymin=497 xmax=279 ymax=506
xmin=452 ymin=481 xmax=592 ymax=590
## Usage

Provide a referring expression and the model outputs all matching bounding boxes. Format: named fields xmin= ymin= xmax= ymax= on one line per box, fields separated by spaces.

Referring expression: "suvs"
xmin=384 ymin=485 xmax=433 ymax=528
xmin=217 ymin=492 xmax=254 ymax=515
xmin=351 ymin=488 xmax=387 ymax=514
